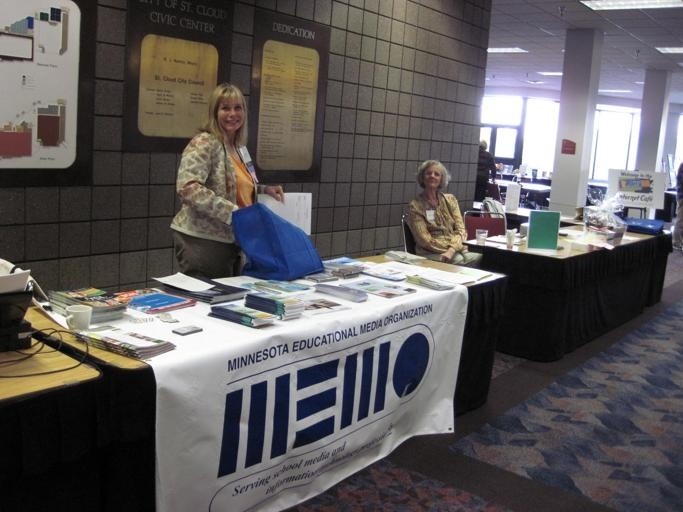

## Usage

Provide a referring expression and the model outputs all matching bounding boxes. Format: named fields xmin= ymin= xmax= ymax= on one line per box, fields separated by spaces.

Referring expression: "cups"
xmin=519 ymin=165 xmax=527 ymax=178
xmin=506 ymin=229 xmax=515 ymax=249
xmin=475 ymin=228 xmax=487 ymax=245
xmin=63 ymin=304 xmax=93 ymax=330
xmin=519 ymin=223 xmax=527 ymax=237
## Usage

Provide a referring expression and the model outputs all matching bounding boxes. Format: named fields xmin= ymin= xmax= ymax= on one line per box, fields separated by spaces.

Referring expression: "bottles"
xmin=531 ymin=169 xmax=537 ymax=178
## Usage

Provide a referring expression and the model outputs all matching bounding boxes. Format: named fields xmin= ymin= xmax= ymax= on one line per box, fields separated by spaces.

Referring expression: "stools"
xmin=622 ymin=204 xmax=647 ymax=219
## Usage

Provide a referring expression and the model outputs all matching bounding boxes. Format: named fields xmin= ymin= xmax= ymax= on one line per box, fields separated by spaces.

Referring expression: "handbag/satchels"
xmin=231 ymin=202 xmax=323 ymax=281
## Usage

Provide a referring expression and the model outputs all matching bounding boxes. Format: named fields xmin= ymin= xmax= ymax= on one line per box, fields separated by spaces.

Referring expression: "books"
xmin=305 ymin=257 xmax=375 ymax=282
xmin=242 ymin=282 xmax=308 ymax=295
xmin=153 ymin=271 xmax=246 ymax=305
xmin=74 ymin=323 xmax=176 ymax=361
xmin=111 ymin=288 xmax=196 ymax=314
xmin=299 ymin=296 xmax=350 ymax=316
xmin=209 ymin=304 xmax=276 ymax=328
xmin=48 ymin=286 xmax=126 ymax=325
xmin=386 ymin=251 xmax=427 ymax=263
xmin=245 ymin=291 xmax=304 ymax=321
xmin=314 ymin=284 xmax=367 ymax=302
xmin=361 ymin=261 xmax=490 ymax=291
xmin=340 ymin=279 xmax=417 ymax=298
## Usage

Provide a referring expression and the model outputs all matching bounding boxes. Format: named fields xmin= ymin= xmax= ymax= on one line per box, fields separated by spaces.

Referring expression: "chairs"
xmin=486 ymin=182 xmax=505 ymax=204
xmin=402 ymin=215 xmax=417 ymax=255
xmin=464 ymin=209 xmax=507 ymax=241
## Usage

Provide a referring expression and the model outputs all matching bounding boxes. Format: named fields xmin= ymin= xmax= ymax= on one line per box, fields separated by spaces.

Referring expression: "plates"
xmin=624 ymin=217 xmax=664 ymax=235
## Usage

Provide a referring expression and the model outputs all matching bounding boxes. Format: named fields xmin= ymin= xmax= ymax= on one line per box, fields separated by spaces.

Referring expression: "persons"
xmin=671 ymin=161 xmax=682 ymax=249
xmin=408 ymin=159 xmax=484 ymax=265
xmin=473 ymin=140 xmax=498 ymax=216
xmin=170 ymin=81 xmax=285 ymax=281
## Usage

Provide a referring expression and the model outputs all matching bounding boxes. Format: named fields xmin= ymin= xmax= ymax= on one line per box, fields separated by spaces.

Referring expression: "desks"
xmin=655 ymin=190 xmax=677 ymax=223
xmin=462 ymin=201 xmax=675 ymax=364
xmin=0 ymin=337 xmax=103 ymax=511
xmin=23 ymin=251 xmax=508 ymax=511
xmin=489 ymin=177 xmax=551 ymax=208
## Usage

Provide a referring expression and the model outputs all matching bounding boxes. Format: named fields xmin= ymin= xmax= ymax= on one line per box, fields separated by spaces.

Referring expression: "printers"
xmin=0 ymin=289 xmax=33 ymax=350
